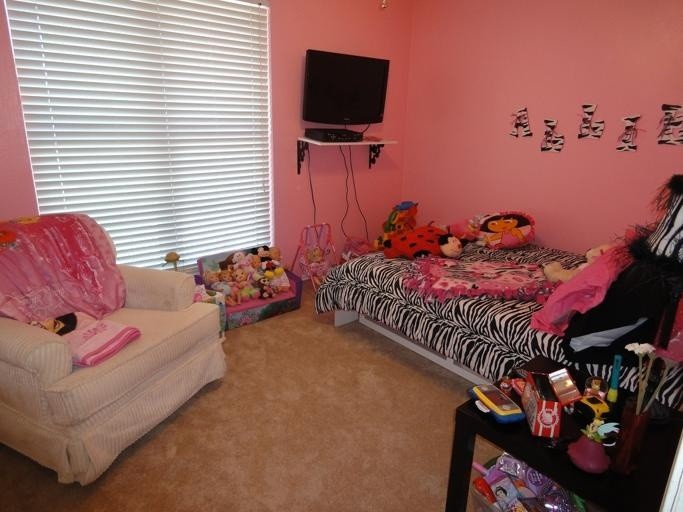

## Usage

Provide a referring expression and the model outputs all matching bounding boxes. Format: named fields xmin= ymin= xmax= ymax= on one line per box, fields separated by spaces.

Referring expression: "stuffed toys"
xmin=544 ymin=243 xmax=611 ymax=286
xmin=384 ymin=223 xmax=463 ymax=258
xmin=373 ymin=199 xmax=417 ymax=250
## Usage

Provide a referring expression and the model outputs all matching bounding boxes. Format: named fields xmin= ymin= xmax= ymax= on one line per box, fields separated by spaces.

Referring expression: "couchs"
xmin=1 ymin=212 xmax=226 ymax=486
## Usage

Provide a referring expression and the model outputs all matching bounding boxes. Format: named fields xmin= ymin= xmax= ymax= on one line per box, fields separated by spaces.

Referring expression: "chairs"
xmin=194 ymin=245 xmax=302 ymax=331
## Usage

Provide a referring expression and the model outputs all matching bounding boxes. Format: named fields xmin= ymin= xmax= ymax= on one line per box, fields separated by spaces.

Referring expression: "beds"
xmin=333 ymin=244 xmax=683 ymax=410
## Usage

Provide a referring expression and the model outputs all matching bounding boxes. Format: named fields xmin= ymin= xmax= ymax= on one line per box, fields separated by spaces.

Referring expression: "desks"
xmin=445 ymin=355 xmax=682 ymax=512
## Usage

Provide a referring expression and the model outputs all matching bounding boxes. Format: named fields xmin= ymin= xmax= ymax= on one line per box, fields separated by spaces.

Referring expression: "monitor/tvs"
xmin=302 ymin=49 xmax=390 ymax=126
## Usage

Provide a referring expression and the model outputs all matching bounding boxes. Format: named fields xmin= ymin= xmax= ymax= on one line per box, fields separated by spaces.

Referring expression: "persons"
xmin=495 ymin=486 xmax=512 ymax=503
xmin=194 ymin=246 xmax=291 ymax=311
